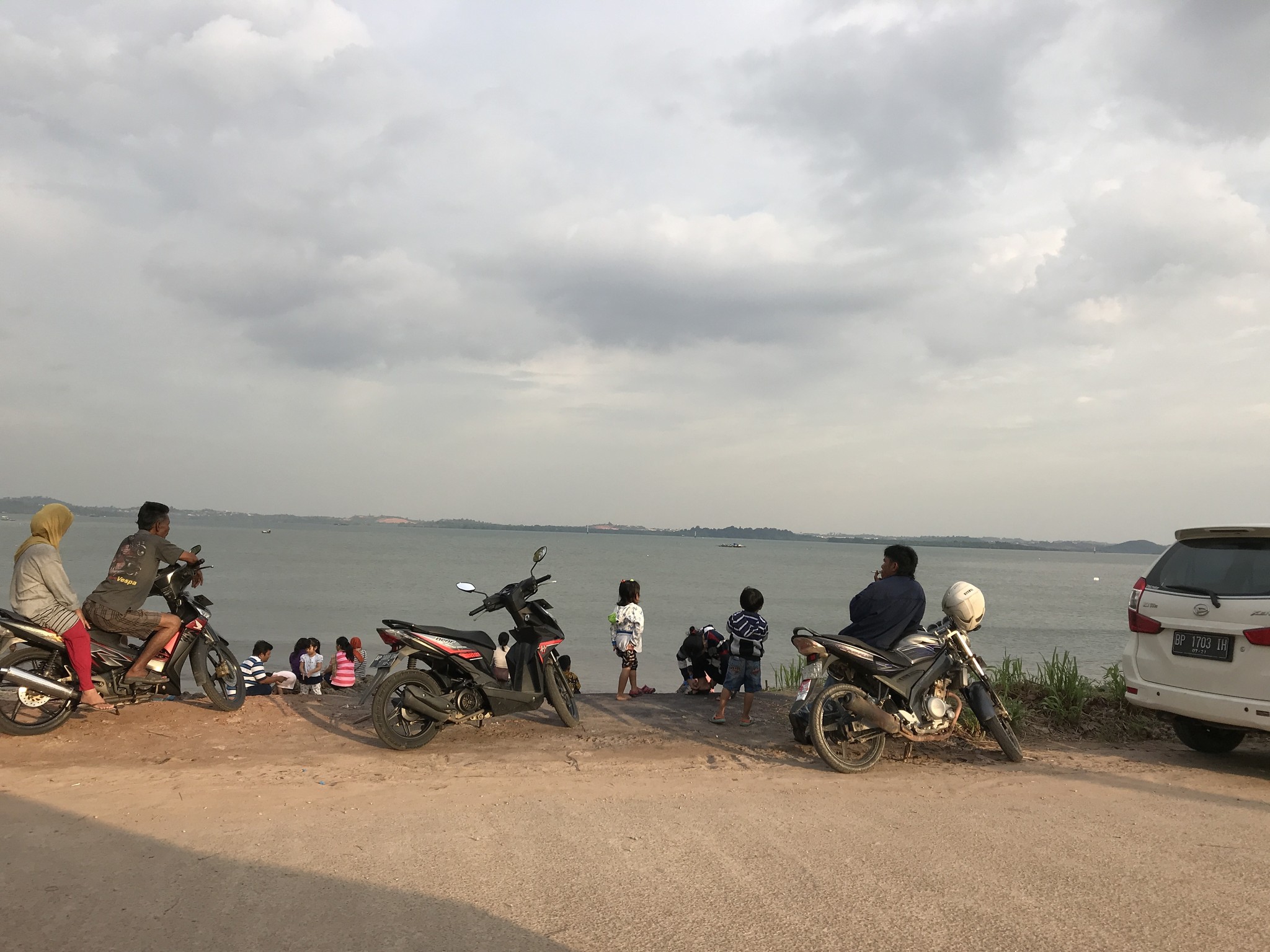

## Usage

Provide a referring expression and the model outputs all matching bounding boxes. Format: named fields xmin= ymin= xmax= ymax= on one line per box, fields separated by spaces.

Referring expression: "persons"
xmin=266 ymin=637 xmax=367 ymax=695
xmin=10 ymin=503 xmax=117 ymax=712
xmin=795 ymin=544 xmax=926 ymax=746
xmin=676 ymin=624 xmax=737 ymax=700
xmin=491 ymin=631 xmax=512 ymax=680
xmin=709 ymin=586 xmax=768 ymax=727
xmin=608 ymin=579 xmax=644 ymax=700
xmin=226 ymin=641 xmax=288 ymax=697
xmin=82 ymin=502 xmax=203 ymax=683
xmin=558 ymin=655 xmax=581 ymax=695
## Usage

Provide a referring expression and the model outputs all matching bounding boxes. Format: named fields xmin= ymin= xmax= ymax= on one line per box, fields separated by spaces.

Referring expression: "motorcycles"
xmin=791 ymin=612 xmax=1024 ymax=774
xmin=358 ymin=546 xmax=580 ymax=752
xmin=0 ymin=544 xmax=246 ymax=737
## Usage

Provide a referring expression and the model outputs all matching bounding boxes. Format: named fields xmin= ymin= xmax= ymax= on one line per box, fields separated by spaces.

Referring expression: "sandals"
xmin=629 ymin=687 xmax=645 ymax=697
xmin=641 ymin=685 xmax=655 ymax=693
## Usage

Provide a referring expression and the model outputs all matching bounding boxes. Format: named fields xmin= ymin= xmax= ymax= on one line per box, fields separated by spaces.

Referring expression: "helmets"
xmin=942 ymin=581 xmax=985 ymax=631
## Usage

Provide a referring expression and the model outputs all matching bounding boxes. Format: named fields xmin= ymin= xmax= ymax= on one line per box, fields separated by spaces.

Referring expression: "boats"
xmin=1 ymin=516 xmax=10 ymax=520
xmin=10 ymin=519 xmax=15 ymax=521
xmin=2 ymin=511 xmax=7 ymax=514
xmin=718 ymin=542 xmax=746 ymax=548
xmin=261 ymin=529 xmax=271 ymax=534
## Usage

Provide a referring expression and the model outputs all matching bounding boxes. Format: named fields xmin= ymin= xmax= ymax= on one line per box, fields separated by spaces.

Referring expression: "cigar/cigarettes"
xmin=870 ymin=571 xmax=881 ymax=573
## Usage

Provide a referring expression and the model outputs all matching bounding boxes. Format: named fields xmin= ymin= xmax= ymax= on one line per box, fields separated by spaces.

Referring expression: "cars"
xmin=1122 ymin=524 xmax=1270 ymax=753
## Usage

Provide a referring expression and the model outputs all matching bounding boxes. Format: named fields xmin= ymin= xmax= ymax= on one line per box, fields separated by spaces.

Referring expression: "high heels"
xmin=716 ymin=691 xmax=737 ymax=701
xmin=685 ymin=688 xmax=711 ymax=695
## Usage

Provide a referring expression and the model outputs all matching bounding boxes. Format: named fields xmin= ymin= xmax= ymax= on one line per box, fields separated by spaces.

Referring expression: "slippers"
xmin=77 ymin=700 xmax=117 ymax=711
xmin=740 ymin=716 xmax=756 ymax=726
xmin=123 ymin=671 xmax=170 ymax=684
xmin=709 ymin=712 xmax=726 ymax=723
